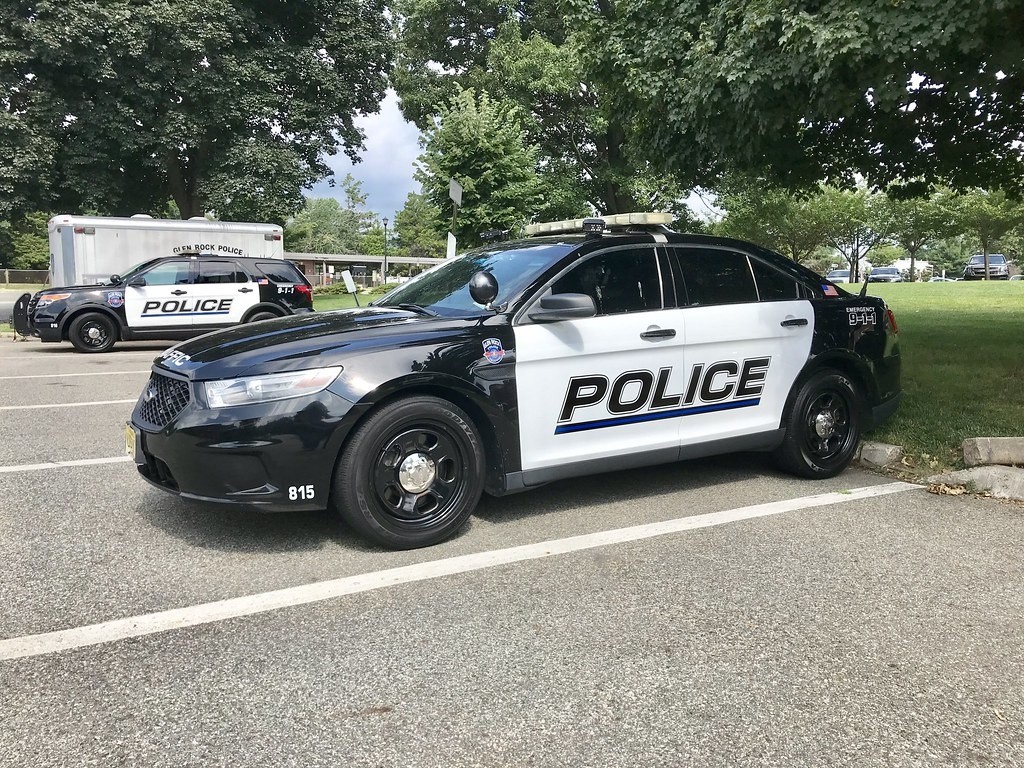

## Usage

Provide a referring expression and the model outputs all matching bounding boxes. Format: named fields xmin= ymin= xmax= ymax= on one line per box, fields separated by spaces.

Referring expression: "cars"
xmin=927 ymin=277 xmax=956 ymax=282
xmin=825 ymin=269 xmax=861 ymax=284
xmin=125 ymin=212 xmax=901 ymax=552
xmin=865 ymin=267 xmax=902 ymax=283
xmin=961 ymin=253 xmax=1009 ymax=281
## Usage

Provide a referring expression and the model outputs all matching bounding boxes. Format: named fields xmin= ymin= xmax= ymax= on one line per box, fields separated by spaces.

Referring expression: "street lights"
xmin=381 ymin=216 xmax=388 ymax=284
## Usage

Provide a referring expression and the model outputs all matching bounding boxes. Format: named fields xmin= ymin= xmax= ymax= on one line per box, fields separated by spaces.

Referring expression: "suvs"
xmin=25 ymin=249 xmax=316 ymax=353
